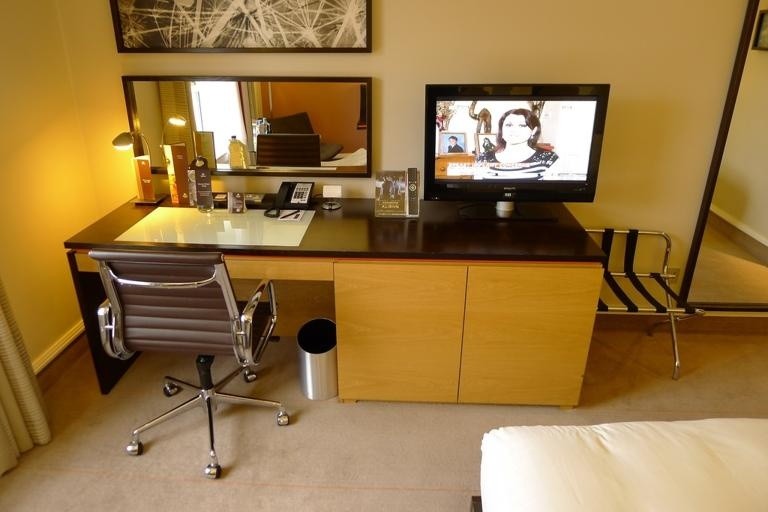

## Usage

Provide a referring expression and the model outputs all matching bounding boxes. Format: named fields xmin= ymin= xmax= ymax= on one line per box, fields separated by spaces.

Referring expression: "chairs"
xmin=254 ymin=133 xmax=321 ymax=167
xmin=583 ymin=226 xmax=705 ymax=379
xmin=89 ymin=247 xmax=291 ymax=480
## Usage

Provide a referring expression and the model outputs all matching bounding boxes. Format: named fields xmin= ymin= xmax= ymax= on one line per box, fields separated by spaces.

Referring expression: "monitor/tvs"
xmin=424 ymin=84 xmax=610 ymax=221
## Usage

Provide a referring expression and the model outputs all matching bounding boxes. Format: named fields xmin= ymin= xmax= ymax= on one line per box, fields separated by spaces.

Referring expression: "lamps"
xmin=160 ymin=113 xmax=187 ymax=167
xmin=112 ymin=131 xmax=168 ymax=206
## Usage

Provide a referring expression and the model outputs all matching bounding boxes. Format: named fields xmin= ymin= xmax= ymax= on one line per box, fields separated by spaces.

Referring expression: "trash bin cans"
xmin=296 ymin=317 xmax=337 ymax=400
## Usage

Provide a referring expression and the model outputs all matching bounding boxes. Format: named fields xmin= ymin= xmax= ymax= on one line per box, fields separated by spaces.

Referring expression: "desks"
xmin=61 ymin=193 xmax=605 ymax=411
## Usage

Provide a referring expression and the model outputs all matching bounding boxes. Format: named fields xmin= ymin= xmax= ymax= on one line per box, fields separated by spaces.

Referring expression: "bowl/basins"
xmin=495 ymin=201 xmax=514 ymax=218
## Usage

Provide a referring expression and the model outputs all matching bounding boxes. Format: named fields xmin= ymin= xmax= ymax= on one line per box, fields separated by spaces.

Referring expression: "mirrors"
xmin=120 ymin=74 xmax=373 ymax=178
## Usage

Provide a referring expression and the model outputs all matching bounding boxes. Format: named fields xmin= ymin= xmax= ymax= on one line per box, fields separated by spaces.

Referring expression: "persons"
xmin=473 ymin=107 xmax=562 ymax=181
xmin=379 ymin=175 xmax=401 ymax=200
xmin=448 ymin=135 xmax=463 ymax=153
xmin=482 ymin=137 xmax=496 ymax=152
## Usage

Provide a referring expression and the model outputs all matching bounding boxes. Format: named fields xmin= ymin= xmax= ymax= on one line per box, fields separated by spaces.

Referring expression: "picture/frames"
xmin=109 ymin=0 xmax=372 ymax=54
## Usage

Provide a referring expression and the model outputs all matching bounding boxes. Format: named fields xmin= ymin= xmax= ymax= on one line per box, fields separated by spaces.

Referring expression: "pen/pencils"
xmin=280 ymin=210 xmax=300 ymax=219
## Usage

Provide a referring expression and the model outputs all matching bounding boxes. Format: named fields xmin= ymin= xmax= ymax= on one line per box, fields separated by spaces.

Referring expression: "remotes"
xmin=407 ymin=168 xmax=418 ymax=214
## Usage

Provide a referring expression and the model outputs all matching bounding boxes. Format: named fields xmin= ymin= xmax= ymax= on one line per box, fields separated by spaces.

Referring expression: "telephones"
xmin=264 ymin=182 xmax=314 ymax=218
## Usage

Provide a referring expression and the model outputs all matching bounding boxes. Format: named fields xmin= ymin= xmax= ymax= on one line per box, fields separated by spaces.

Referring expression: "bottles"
xmin=229 ymin=135 xmax=243 ymax=169
